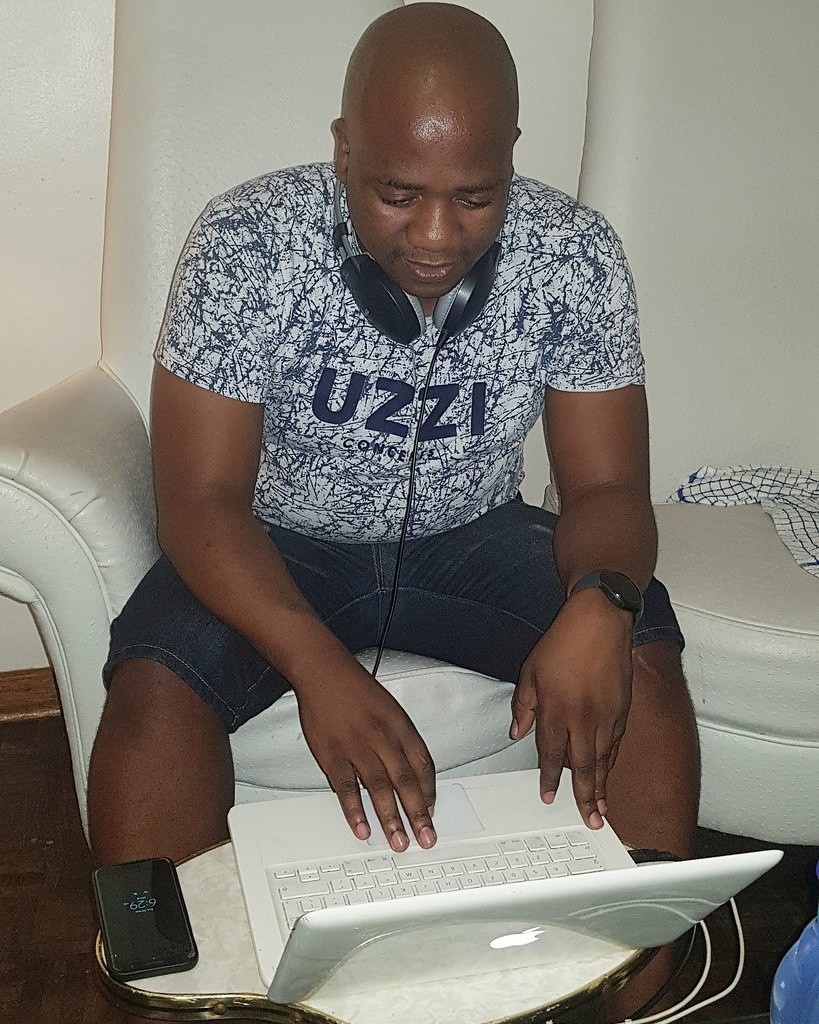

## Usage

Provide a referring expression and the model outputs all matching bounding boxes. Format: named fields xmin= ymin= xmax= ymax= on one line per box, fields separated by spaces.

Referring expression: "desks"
xmin=92 ymin=838 xmax=661 ymax=1024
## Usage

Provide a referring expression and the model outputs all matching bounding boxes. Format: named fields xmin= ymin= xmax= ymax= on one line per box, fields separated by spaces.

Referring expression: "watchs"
xmin=569 ymin=568 xmax=643 ymax=629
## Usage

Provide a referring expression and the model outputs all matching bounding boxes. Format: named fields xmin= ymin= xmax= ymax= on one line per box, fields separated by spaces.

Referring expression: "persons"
xmin=86 ymin=3 xmax=702 ymax=1023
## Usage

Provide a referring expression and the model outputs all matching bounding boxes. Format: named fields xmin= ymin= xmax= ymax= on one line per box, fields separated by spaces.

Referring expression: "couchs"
xmin=0 ymin=0 xmax=819 ymax=849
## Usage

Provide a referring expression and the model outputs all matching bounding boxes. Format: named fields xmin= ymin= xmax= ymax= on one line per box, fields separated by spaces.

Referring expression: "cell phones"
xmin=91 ymin=856 xmax=198 ymax=982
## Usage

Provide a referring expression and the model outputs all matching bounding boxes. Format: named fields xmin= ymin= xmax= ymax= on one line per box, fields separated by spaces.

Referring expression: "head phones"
xmin=334 ymin=178 xmax=501 ymax=345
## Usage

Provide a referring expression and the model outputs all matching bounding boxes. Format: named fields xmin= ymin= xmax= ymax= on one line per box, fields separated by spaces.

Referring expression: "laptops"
xmin=228 ymin=765 xmax=786 ymax=1003
